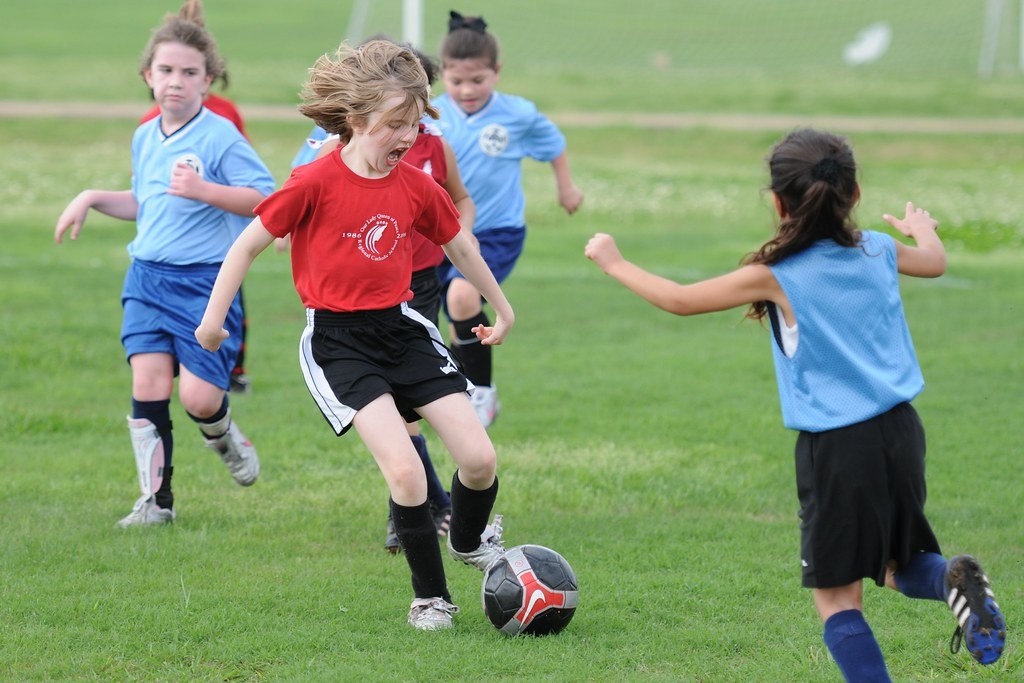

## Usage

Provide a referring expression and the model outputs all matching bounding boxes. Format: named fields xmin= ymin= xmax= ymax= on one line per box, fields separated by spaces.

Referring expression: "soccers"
xmin=480 ymin=544 xmax=581 ymax=639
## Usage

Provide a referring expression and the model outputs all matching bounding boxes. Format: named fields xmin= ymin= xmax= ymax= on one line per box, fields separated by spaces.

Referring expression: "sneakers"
xmin=446 ymin=514 xmax=508 ymax=575
xmin=428 ymin=491 xmax=451 ymax=537
xmin=118 ymin=494 xmax=175 ymax=527
xmin=202 ymin=418 xmax=259 ymax=487
xmin=469 ymin=381 xmax=497 ymax=428
xmin=407 ymin=595 xmax=460 ymax=631
xmin=385 ymin=516 xmax=401 ymax=556
xmin=946 ymin=554 xmax=1006 ymax=664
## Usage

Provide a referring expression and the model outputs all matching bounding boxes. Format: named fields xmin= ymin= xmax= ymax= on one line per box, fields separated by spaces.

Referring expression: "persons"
xmin=584 ymin=128 xmax=1006 ymax=683
xmin=274 ymin=34 xmax=480 ymax=553
xmin=195 ymin=38 xmax=515 ymax=631
xmin=54 ymin=1 xmax=276 ymax=527
xmin=418 ymin=10 xmax=583 ymax=428
